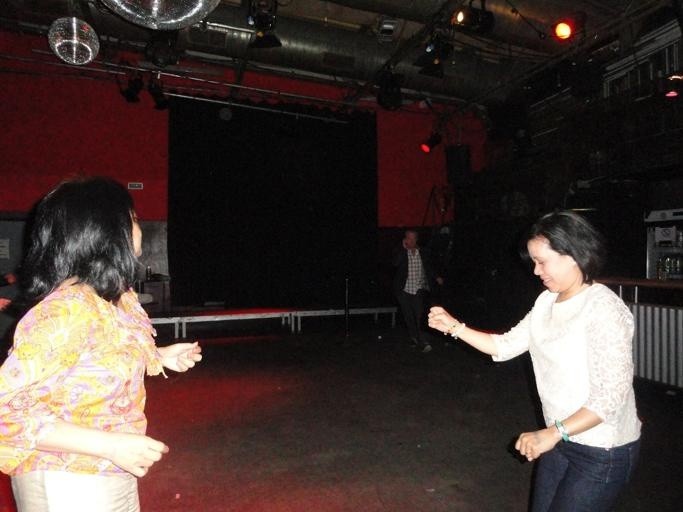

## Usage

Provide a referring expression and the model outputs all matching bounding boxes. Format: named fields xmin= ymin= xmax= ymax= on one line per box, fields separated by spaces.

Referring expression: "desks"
xmin=592 ymin=277 xmax=683 ymax=305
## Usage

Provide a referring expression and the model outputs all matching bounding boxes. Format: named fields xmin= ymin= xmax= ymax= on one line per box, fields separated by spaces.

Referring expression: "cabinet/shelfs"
xmin=145 ymin=266 xmax=152 ymax=282
xmin=659 ymin=257 xmax=681 ymax=275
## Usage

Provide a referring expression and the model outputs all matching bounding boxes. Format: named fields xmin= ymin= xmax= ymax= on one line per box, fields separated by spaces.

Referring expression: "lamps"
xmin=146 ymin=6 xmax=682 ymax=154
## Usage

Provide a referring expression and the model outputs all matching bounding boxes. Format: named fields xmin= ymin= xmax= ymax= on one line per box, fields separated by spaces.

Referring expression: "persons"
xmin=0 ymin=174 xmax=203 ymax=510
xmin=386 ymin=223 xmax=444 ymax=355
xmin=426 ymin=209 xmax=642 ymax=509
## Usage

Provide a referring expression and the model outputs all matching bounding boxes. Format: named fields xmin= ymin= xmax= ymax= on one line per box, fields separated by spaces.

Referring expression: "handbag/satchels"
xmin=448 ymin=322 xmax=469 ymax=340
xmin=553 ymin=419 xmax=570 ymax=443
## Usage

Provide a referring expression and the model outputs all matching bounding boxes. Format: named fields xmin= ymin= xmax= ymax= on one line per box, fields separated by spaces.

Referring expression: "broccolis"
xmin=645 ymin=209 xmax=682 ymax=282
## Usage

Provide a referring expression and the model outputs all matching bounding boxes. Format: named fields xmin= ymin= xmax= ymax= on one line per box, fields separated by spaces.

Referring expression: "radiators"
xmin=620 ymin=300 xmax=683 ymax=396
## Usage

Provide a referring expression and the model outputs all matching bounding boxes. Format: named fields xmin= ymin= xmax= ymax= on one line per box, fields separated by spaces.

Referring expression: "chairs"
xmin=409 ymin=344 xmax=432 ymax=354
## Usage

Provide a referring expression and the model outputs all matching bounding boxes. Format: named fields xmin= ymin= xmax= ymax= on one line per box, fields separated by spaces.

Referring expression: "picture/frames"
xmin=592 ymin=277 xmax=683 ymax=305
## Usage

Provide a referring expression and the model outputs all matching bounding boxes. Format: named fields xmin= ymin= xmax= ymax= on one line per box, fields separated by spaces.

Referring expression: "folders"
xmin=620 ymin=300 xmax=683 ymax=396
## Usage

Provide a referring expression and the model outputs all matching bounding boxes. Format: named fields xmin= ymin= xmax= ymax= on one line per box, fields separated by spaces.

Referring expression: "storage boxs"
xmin=147 ymin=301 xmax=398 ymax=347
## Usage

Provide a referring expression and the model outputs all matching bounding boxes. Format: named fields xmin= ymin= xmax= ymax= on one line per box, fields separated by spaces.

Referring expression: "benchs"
xmin=147 ymin=301 xmax=398 ymax=347
